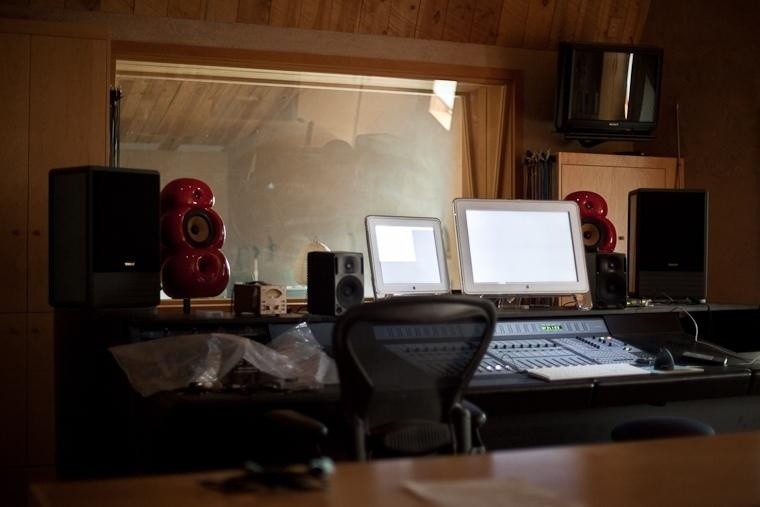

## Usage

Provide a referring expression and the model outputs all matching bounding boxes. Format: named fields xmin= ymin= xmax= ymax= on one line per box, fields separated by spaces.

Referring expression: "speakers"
xmin=306 ymin=249 xmax=364 ymax=317
xmin=627 ymin=187 xmax=707 ymax=304
xmin=591 ymin=252 xmax=627 ymax=309
xmin=47 ymin=165 xmax=162 ymax=311
xmin=161 ymin=177 xmax=231 ymax=299
xmin=563 ymin=190 xmax=616 ymax=258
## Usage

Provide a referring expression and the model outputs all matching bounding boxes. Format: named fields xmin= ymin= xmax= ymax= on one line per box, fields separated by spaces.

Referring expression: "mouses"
xmin=654 ymin=349 xmax=674 ymax=371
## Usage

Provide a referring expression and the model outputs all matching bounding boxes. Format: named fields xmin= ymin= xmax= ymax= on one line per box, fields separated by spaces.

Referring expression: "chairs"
xmin=332 ymin=295 xmax=496 ymax=460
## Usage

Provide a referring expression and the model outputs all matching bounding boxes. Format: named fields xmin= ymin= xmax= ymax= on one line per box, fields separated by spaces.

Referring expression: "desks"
xmin=153 ymin=338 xmax=760 ymax=408
xmin=29 ymin=432 xmax=760 ymax=507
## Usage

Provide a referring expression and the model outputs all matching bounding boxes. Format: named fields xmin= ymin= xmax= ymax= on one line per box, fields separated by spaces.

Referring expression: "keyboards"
xmin=526 ymin=362 xmax=650 ymax=380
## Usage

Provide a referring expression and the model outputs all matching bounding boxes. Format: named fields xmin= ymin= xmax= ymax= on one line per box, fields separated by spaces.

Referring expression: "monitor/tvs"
xmin=553 ymin=39 xmax=664 ymax=135
xmin=453 ymin=196 xmax=590 ymax=310
xmin=364 ymin=214 xmax=450 ymax=299
